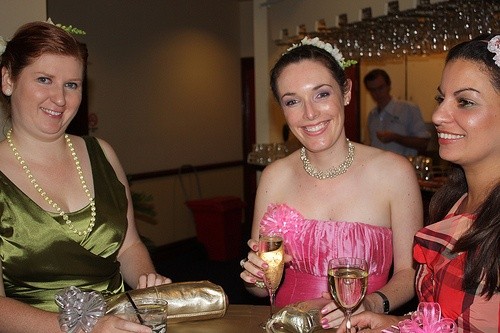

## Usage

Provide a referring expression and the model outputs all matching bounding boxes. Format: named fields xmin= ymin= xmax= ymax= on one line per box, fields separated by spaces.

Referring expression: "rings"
xmin=242 ymin=260 xmax=246 ymax=267
xmin=353 ymin=326 xmax=358 ymax=333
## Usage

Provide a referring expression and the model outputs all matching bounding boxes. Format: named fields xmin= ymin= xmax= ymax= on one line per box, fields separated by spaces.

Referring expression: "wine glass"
xmin=258 ymin=232 xmax=284 ymax=329
xmin=328 ymin=257 xmax=369 ymax=333
xmin=327 ymin=0 xmax=500 ymax=63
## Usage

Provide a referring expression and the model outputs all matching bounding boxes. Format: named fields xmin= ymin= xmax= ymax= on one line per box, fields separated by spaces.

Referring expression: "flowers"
xmin=282 ymin=34 xmax=357 ymax=70
xmin=1 ymin=17 xmax=86 ymax=55
xmin=487 ymin=34 xmax=500 ymax=67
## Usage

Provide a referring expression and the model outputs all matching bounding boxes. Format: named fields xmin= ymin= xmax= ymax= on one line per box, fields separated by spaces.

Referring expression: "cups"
xmin=248 ymin=143 xmax=290 ymax=166
xmin=123 ymin=298 xmax=169 ymax=333
xmin=408 ymin=155 xmax=434 ymax=180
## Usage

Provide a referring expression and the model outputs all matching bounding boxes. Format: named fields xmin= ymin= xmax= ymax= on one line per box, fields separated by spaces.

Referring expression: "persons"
xmin=0 ymin=21 xmax=172 ymax=333
xmin=336 ymin=33 xmax=500 ymax=333
xmin=240 ymin=45 xmax=424 ymax=330
xmin=363 ymin=69 xmax=432 ymax=157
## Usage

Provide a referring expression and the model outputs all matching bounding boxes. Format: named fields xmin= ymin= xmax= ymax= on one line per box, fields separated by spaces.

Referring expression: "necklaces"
xmin=6 ymin=128 xmax=96 ymax=236
xmin=300 ymin=138 xmax=354 ymax=179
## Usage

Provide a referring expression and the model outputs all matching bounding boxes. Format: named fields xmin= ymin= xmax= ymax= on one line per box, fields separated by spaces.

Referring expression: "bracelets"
xmin=373 ymin=291 xmax=390 ymax=312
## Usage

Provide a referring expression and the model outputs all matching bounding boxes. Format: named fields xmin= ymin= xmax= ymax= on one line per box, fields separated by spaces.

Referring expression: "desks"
xmin=164 ymin=303 xmax=339 ymax=333
